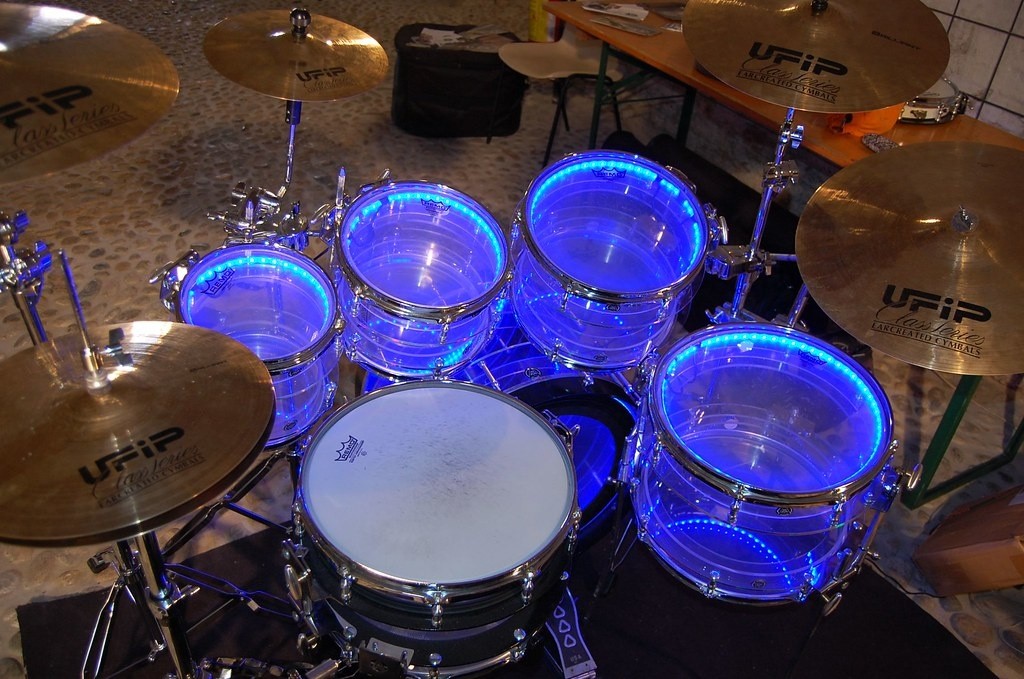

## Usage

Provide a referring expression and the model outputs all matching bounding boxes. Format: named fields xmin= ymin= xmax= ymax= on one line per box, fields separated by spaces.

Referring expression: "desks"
xmin=542 ymin=0 xmax=1024 ymax=171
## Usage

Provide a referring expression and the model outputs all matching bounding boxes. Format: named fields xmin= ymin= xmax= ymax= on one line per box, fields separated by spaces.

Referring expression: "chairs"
xmin=498 ymin=21 xmax=631 ymax=169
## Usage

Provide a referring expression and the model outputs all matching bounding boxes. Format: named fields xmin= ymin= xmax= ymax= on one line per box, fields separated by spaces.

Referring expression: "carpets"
xmin=13 ymin=497 xmax=1005 ymax=679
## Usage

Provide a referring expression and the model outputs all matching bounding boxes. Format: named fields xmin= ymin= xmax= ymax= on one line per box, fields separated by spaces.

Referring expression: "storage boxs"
xmin=391 ymin=22 xmax=530 ymax=143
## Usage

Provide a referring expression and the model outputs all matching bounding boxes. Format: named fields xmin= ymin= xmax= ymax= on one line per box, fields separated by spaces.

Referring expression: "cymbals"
xmin=0 ymin=2 xmax=181 ymax=189
xmin=0 ymin=317 xmax=281 ymax=551
xmin=793 ymin=139 xmax=1024 ymax=378
xmin=679 ymin=0 xmax=953 ymax=116
xmin=200 ymin=5 xmax=391 ymax=105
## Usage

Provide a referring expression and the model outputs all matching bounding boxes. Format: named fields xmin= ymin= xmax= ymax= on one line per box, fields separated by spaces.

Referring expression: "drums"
xmin=354 ymin=284 xmax=644 ymax=560
xmin=176 ymin=241 xmax=344 ymax=459
xmin=290 ymin=376 xmax=580 ymax=679
xmin=330 ymin=178 xmax=513 ymax=386
xmin=513 ymin=144 xmax=712 ymax=379
xmin=633 ymin=322 xmax=895 ymax=610
